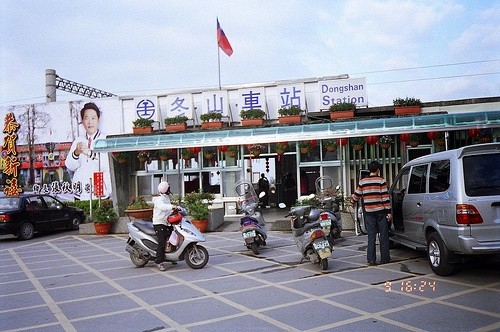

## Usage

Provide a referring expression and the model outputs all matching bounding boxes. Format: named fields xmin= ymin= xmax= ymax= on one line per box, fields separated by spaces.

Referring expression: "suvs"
xmin=355 ymin=142 xmax=500 ymax=278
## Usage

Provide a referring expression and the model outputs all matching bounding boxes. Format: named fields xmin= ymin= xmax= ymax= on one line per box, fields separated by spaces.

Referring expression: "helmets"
xmin=308 ymin=208 xmax=320 ymax=222
xmin=158 ymin=181 xmax=170 ymax=193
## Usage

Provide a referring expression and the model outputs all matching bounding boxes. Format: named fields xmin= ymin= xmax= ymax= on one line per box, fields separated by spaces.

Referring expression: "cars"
xmin=1 ymin=192 xmax=87 ymax=240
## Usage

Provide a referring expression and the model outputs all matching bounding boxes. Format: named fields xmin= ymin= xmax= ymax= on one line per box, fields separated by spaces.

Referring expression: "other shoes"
xmin=158 ymin=262 xmax=166 ymax=271
xmin=380 ymin=259 xmax=390 ymax=264
xmin=367 ymin=261 xmax=375 ymax=265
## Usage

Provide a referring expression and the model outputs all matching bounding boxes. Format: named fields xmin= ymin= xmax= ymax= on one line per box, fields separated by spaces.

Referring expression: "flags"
xmin=217 ymin=17 xmax=233 ymax=57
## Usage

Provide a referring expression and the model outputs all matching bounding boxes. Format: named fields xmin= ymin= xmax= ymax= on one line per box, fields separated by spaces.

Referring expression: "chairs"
xmin=30 ymin=201 xmax=42 ymax=209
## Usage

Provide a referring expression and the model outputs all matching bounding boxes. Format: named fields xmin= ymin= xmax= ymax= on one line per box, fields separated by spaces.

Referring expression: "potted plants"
xmin=228 ymin=146 xmax=238 ymax=157
xmin=408 ymin=133 xmax=417 ymax=147
xmin=275 ymin=144 xmax=286 ymax=156
xmin=325 ymin=140 xmax=337 ymax=152
xmin=251 ymin=146 xmax=261 ymax=157
xmin=434 ymin=134 xmax=445 ymax=145
xmin=380 ymin=136 xmax=394 ymax=148
xmin=133 ymin=118 xmax=154 ymax=135
xmin=298 ymin=141 xmax=310 ymax=153
xmin=278 ymin=106 xmax=304 ymax=124
xmin=353 ymin=137 xmax=365 ymax=151
xmin=480 ymin=130 xmax=493 ymax=143
xmin=199 ymin=112 xmax=223 ymax=130
xmin=116 ymin=146 xmax=217 ymax=163
xmin=329 ymin=102 xmax=356 ymax=120
xmin=164 ymin=116 xmax=188 ymax=132
xmin=240 ymin=109 xmax=265 ymax=127
xmin=393 ymin=98 xmax=421 ymax=117
xmin=90 ymin=192 xmax=211 ymax=233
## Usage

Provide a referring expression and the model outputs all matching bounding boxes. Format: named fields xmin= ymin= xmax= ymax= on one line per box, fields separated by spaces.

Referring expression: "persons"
xmin=258 ymin=172 xmax=270 ymax=206
xmin=350 ymin=161 xmax=393 ymax=267
xmin=64 ymin=102 xmax=112 ymax=203
xmin=151 ymin=181 xmax=189 ymax=271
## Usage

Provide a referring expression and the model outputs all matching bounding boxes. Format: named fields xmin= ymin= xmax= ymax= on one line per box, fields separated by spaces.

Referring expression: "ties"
xmin=86 ymin=137 xmax=91 ymax=161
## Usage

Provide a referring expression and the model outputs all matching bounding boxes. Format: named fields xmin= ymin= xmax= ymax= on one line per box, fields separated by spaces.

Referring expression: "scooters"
xmin=125 ymin=201 xmax=209 ymax=270
xmin=279 ymin=194 xmax=334 ymax=271
xmin=234 ymin=180 xmax=270 ymax=254
xmin=318 ymin=185 xmax=344 ymax=245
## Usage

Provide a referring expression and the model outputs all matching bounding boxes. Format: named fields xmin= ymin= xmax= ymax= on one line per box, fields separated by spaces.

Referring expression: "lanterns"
xmin=219 ymin=146 xmax=227 ymax=152
xmin=366 ymin=136 xmax=379 ymax=145
xmin=428 ymin=131 xmax=440 ymax=140
xmin=189 ymin=147 xmax=201 ymax=156
xmin=400 ymin=134 xmax=410 ymax=142
xmin=336 ymin=138 xmax=347 ymax=146
xmin=311 ymin=140 xmax=318 ymax=147
xmin=467 ymin=129 xmax=481 ymax=137
xmin=280 ymin=142 xmax=288 ymax=147
